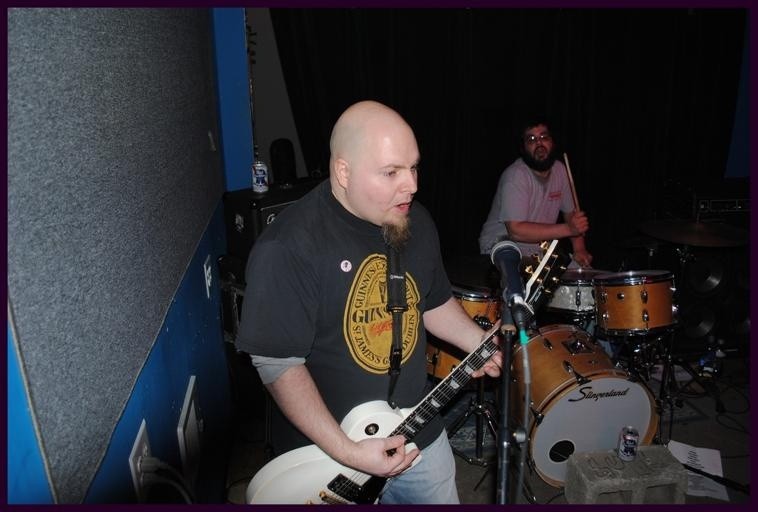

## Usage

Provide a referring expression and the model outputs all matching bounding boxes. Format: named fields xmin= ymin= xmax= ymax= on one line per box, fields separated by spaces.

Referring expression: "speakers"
xmin=636 ymin=221 xmax=750 ymax=353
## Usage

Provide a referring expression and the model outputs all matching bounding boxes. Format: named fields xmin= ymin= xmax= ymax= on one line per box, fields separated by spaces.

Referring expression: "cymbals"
xmin=638 ymin=219 xmax=748 ymax=248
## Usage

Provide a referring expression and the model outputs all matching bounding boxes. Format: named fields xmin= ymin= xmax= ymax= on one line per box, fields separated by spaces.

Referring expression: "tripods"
xmin=650 ymin=273 xmax=730 ymax=417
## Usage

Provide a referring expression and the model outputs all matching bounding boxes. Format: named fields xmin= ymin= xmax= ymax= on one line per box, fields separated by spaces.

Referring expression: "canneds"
xmin=251 ymin=162 xmax=270 ymax=193
xmin=617 ymin=427 xmax=639 ymax=462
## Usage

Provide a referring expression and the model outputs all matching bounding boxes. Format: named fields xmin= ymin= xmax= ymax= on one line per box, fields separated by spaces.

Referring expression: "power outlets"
xmin=128 ymin=420 xmax=155 ymax=499
xmin=203 ymin=257 xmax=213 ymax=299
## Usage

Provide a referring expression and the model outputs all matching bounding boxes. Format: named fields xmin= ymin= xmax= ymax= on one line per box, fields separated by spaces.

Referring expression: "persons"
xmin=233 ymin=100 xmax=513 ymax=506
xmin=478 ymin=103 xmax=593 ymax=305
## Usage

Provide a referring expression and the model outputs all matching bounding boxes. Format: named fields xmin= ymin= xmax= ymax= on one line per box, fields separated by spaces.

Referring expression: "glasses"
xmin=526 ymin=134 xmax=551 ymax=143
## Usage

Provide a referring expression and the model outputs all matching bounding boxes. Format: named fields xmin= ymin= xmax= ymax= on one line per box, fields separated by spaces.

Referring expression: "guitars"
xmin=247 ymin=238 xmax=574 ymax=505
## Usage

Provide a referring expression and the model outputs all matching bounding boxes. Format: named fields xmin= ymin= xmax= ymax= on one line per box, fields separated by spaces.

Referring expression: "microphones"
xmin=489 ymin=241 xmax=525 ymax=325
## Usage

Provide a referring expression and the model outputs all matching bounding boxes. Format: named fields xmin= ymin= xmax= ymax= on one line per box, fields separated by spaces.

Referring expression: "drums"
xmin=424 ymin=286 xmax=500 ymax=381
xmin=547 ymin=269 xmax=612 ymax=315
xmin=597 ymin=267 xmax=678 ymax=337
xmin=510 ymin=326 xmax=658 ymax=489
